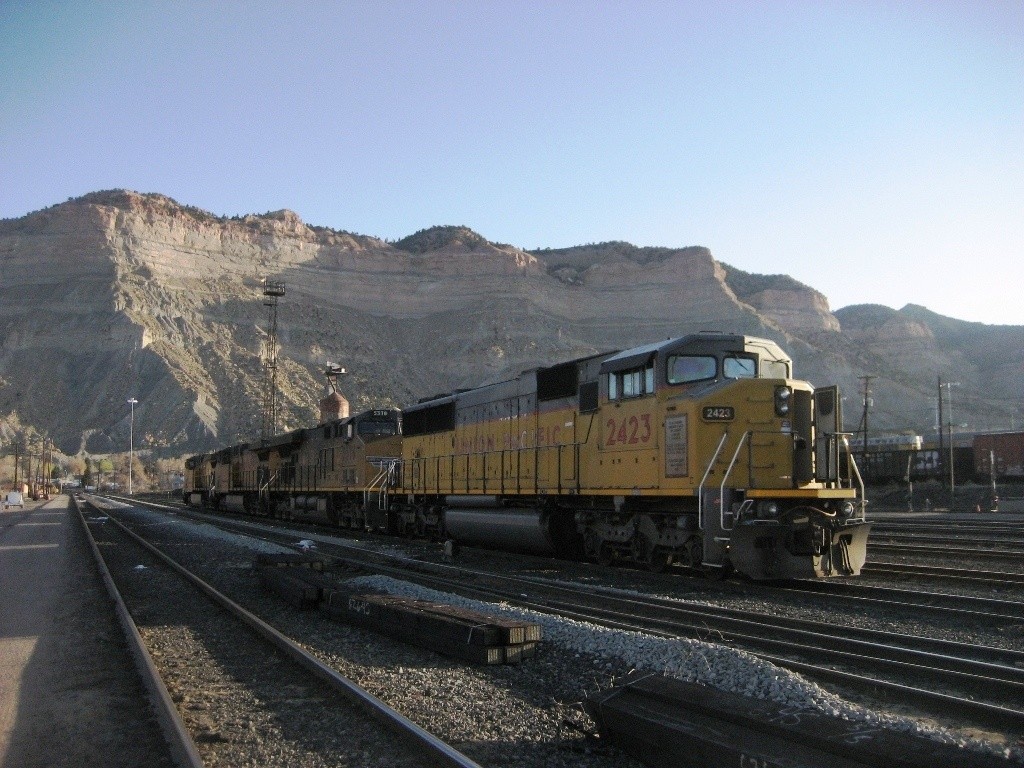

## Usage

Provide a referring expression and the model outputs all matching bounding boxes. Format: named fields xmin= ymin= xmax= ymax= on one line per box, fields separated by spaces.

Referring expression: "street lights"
xmin=127 ymin=397 xmax=138 ymax=495
xmin=940 ymin=382 xmax=962 ymax=491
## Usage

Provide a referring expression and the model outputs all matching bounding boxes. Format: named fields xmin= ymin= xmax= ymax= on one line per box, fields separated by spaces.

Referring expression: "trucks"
xmin=4 ymin=492 xmax=24 ymax=510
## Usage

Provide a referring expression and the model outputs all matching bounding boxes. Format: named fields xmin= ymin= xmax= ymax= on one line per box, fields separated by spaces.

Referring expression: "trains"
xmin=838 ymin=425 xmax=1024 ymax=488
xmin=179 ymin=330 xmax=876 ymax=581
